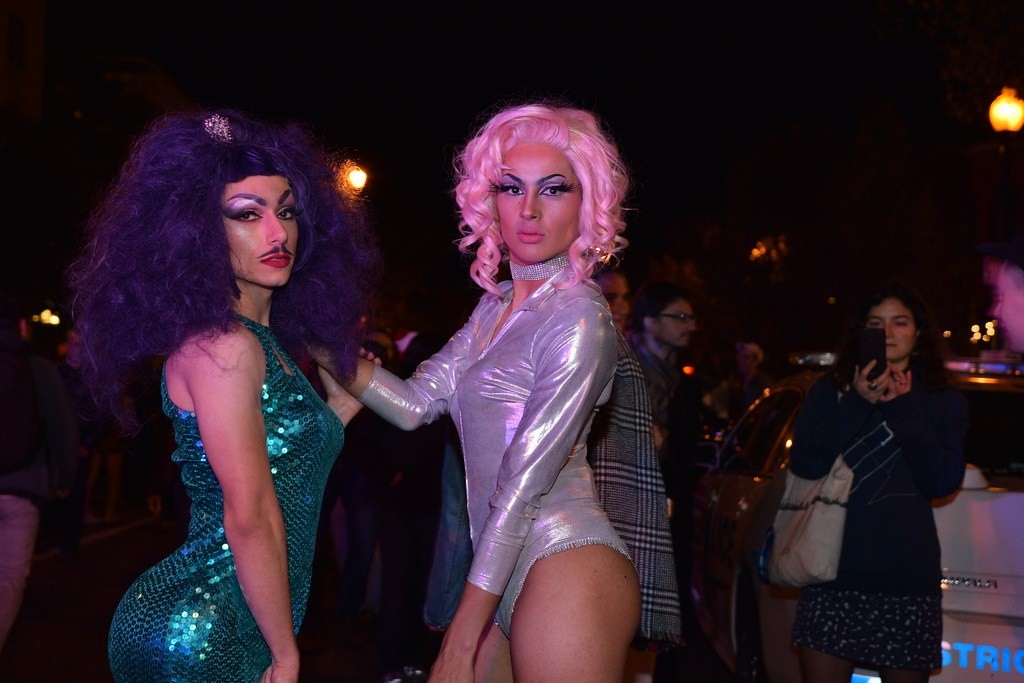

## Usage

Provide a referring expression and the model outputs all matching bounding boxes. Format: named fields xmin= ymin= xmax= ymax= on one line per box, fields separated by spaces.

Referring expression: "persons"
xmin=0 ymin=298 xmax=183 ymax=649
xmin=306 ymin=100 xmax=640 ymax=683
xmin=790 ymin=292 xmax=968 ymax=683
xmin=987 ymin=248 xmax=1024 ymax=351
xmin=589 ymin=266 xmax=772 ymax=683
xmin=307 ymin=328 xmax=475 ymax=683
xmin=66 ymin=111 xmax=383 ymax=683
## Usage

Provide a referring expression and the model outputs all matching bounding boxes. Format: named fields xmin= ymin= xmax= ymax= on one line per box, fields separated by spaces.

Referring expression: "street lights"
xmin=991 ymin=86 xmax=1024 ymax=228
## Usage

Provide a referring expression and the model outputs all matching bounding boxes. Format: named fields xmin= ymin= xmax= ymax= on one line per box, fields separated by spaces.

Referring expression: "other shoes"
xmin=382 ymin=670 xmax=407 ymax=683
xmin=401 ymin=662 xmax=427 ymax=683
xmin=100 ymin=514 xmax=130 ymax=527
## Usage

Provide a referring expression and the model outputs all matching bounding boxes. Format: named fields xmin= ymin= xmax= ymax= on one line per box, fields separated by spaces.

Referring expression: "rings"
xmin=895 ymin=377 xmax=900 ymax=382
xmin=868 ymin=382 xmax=878 ymax=391
xmin=900 ymin=381 xmax=906 ymax=385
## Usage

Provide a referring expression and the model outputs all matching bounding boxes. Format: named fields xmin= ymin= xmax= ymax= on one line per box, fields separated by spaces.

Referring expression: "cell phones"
xmin=854 ymin=326 xmax=887 ymax=382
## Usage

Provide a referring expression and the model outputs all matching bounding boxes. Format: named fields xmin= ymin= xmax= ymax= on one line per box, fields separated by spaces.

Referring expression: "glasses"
xmin=783 ymin=364 xmax=831 ymax=373
xmin=653 ymin=313 xmax=695 ymax=321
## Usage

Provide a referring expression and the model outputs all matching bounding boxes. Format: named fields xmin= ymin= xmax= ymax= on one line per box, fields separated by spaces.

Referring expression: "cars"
xmin=682 ymin=347 xmax=1024 ymax=683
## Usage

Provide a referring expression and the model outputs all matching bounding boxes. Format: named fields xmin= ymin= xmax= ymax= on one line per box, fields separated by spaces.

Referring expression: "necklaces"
xmin=508 ymin=253 xmax=571 ymax=280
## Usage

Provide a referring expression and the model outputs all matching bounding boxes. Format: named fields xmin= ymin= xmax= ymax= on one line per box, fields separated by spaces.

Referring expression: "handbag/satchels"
xmin=765 ymin=452 xmax=856 ymax=588
